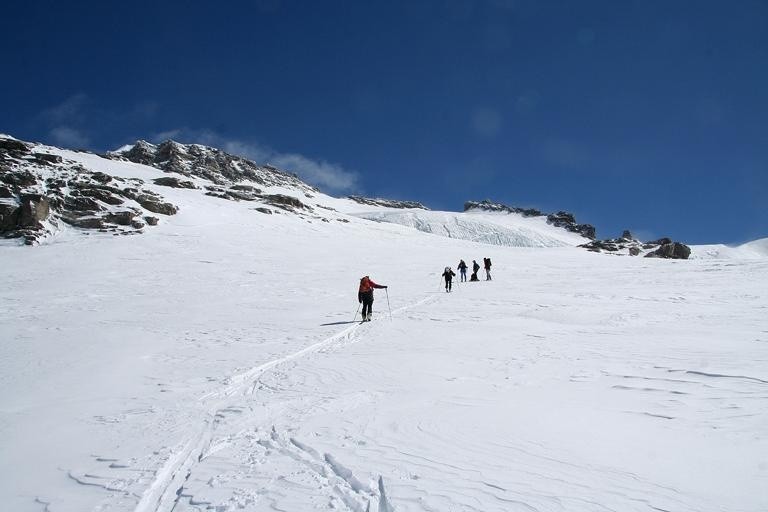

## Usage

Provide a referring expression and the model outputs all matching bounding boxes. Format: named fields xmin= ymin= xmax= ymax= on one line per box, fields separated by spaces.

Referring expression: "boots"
xmin=362 ymin=312 xmax=373 ymax=321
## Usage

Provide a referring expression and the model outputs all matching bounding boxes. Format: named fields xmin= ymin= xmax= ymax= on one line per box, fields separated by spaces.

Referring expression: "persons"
xmin=457 ymin=259 xmax=469 ymax=283
xmin=472 ymin=259 xmax=481 ymax=281
xmin=483 ymin=257 xmax=492 ymax=281
xmin=441 ymin=266 xmax=457 ymax=293
xmin=357 ymin=275 xmax=388 ymax=321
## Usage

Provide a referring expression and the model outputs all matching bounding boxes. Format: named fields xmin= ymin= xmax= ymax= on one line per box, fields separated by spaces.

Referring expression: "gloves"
xmin=384 ymin=286 xmax=387 ymax=288
xmin=359 ymin=297 xmax=363 ymax=303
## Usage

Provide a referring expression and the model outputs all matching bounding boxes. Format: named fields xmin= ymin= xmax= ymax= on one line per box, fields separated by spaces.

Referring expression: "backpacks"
xmin=359 ymin=277 xmax=371 ymax=294
xmin=486 ymin=258 xmax=492 ymax=266
